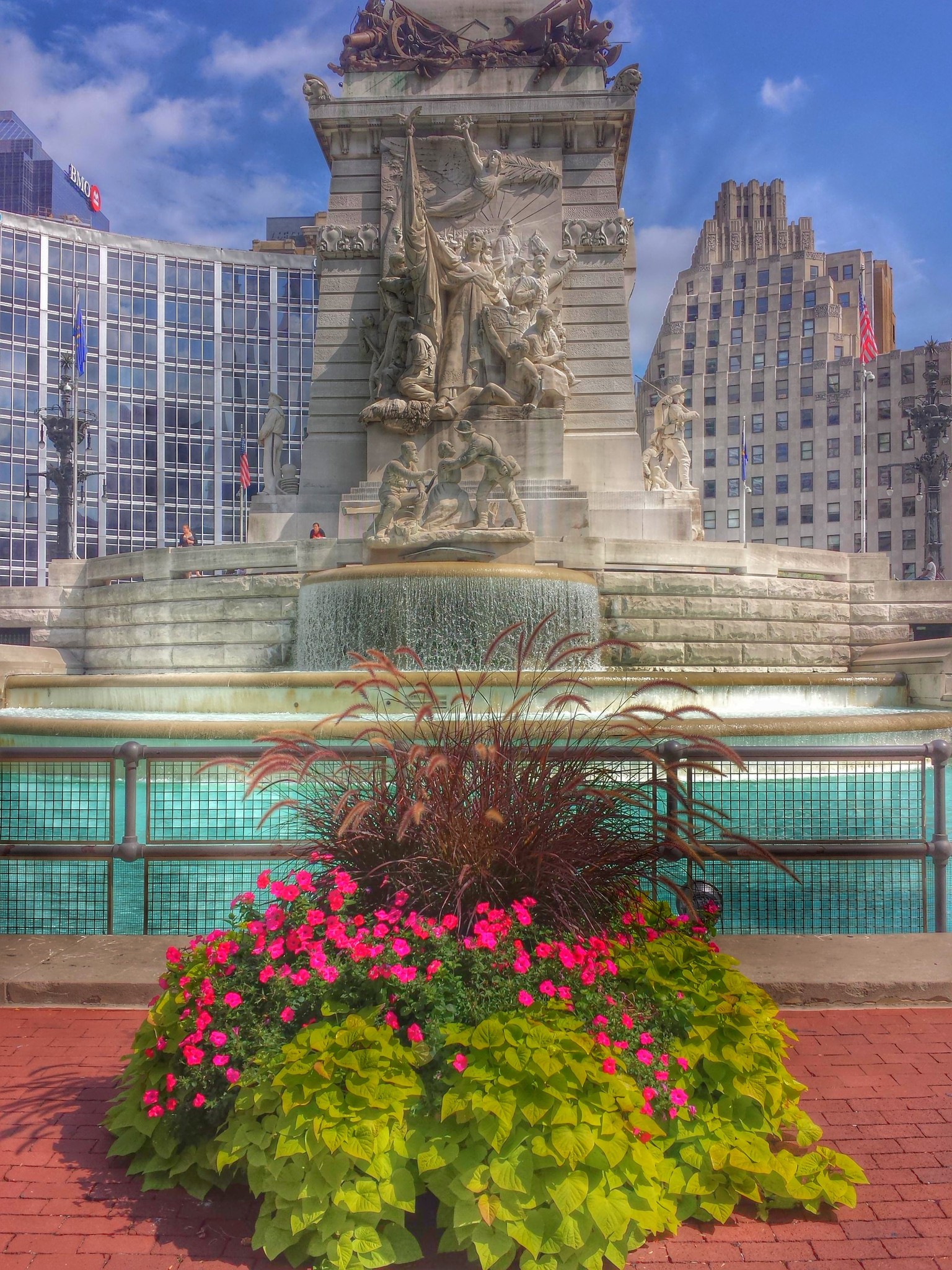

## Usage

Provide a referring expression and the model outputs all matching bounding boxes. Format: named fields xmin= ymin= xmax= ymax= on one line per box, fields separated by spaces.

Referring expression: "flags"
xmin=858 ymin=280 xmax=878 ymax=365
xmin=742 ymin=422 xmax=749 ymax=482
xmin=72 ymin=300 xmax=88 ymax=377
xmin=239 ymin=434 xmax=251 ymax=489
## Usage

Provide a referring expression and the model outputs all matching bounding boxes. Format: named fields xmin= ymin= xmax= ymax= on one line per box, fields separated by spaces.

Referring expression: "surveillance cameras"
xmin=866 ymin=374 xmax=875 ymax=383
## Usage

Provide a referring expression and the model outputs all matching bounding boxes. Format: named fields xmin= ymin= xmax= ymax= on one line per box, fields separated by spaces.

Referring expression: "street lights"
xmin=24 ymin=350 xmax=109 ymax=561
xmin=860 ymin=363 xmax=876 ymax=553
xmin=897 ymin=335 xmax=952 ymax=572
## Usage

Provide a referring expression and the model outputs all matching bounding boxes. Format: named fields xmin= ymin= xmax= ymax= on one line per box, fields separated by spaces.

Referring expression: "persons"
xmin=421 ymin=441 xmax=475 ymax=530
xmin=359 ymin=105 xmax=581 ymax=421
xmin=643 ymin=385 xmax=700 ymax=491
xmin=256 ymin=392 xmax=286 ymax=495
xmin=441 ymin=420 xmax=529 ymax=532
xmin=334 ymin=0 xmax=616 ymax=89
xmin=177 ymin=525 xmax=201 ymax=579
xmin=237 ymin=568 xmax=246 ymax=574
xmin=915 ymin=555 xmax=936 ymax=580
xmin=310 ymin=523 xmax=325 ymax=538
xmin=375 ymin=441 xmax=436 ymax=538
